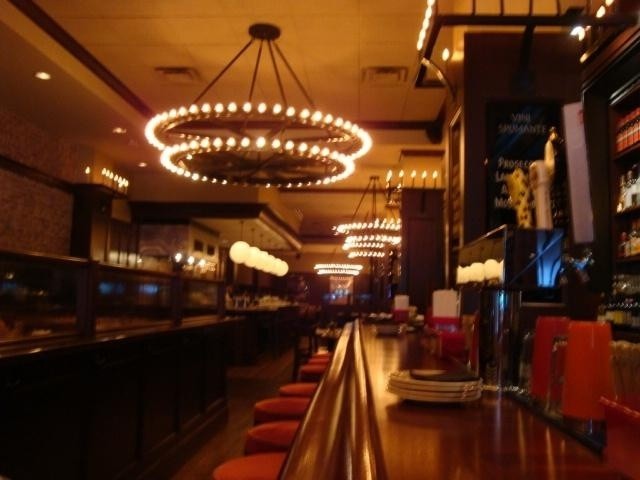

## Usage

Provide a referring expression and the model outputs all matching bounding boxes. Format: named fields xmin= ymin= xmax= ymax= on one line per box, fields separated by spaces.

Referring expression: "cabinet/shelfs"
xmin=576 ymin=17 xmax=640 ymax=336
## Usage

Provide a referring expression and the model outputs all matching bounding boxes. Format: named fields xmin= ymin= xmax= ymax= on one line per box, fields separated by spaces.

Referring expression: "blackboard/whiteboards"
xmin=485 ymin=101 xmax=570 ymax=240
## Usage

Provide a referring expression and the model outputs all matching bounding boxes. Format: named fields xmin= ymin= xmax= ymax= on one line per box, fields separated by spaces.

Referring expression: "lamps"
xmin=314 ymin=240 xmax=362 ymax=275
xmin=229 ymin=219 xmax=290 ymax=276
xmin=337 ymin=176 xmax=402 ymax=261
xmin=145 ymin=24 xmax=372 ymax=187
xmin=173 ymin=252 xmax=206 ymax=268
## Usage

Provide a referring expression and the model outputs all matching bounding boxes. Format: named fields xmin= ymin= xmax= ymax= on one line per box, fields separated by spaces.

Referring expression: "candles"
xmin=85 ymin=165 xmax=130 ymax=195
xmin=386 ymin=169 xmax=438 ymax=190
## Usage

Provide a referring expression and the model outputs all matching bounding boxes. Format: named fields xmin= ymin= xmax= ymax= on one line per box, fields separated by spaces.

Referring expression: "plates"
xmin=385 ymin=371 xmax=484 ymax=402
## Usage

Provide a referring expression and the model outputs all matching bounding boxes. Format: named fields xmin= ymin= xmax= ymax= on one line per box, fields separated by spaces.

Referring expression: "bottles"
xmin=604 ymin=107 xmax=639 ymax=330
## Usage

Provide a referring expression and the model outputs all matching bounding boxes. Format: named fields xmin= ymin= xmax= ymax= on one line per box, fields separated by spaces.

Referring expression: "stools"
xmin=210 ymin=352 xmax=334 ymax=480
